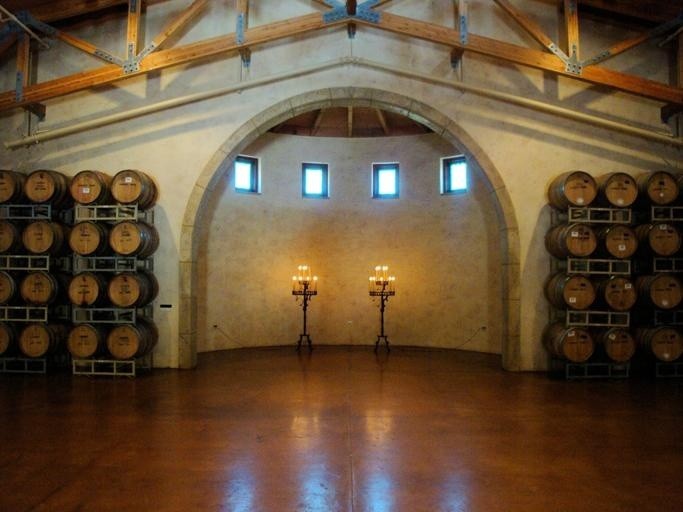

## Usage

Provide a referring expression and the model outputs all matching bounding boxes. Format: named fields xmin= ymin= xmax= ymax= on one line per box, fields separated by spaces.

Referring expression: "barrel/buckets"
xmin=637 ymin=327 xmax=680 ymax=361
xmin=596 ymin=225 xmax=638 ymax=259
xmin=596 ymin=170 xmax=639 ymax=209
xmin=542 ymin=273 xmax=597 ymax=311
xmin=634 ymin=169 xmax=680 ymax=207
xmin=0 ymin=167 xmax=159 ymax=361
xmin=543 ymin=224 xmax=597 ymax=260
xmin=545 ymin=170 xmax=597 ymax=212
xmin=593 ymin=328 xmax=635 ymax=363
xmin=541 ymin=321 xmax=594 ymax=362
xmin=637 ymin=274 xmax=681 ymax=309
xmin=596 ymin=277 xmax=637 ymax=312
xmin=634 ymin=223 xmax=679 ymax=256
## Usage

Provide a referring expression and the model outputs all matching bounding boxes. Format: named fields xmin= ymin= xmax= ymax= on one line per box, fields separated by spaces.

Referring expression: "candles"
xmin=291 ymin=264 xmax=317 ymax=290
xmin=366 ymin=264 xmax=395 ymax=293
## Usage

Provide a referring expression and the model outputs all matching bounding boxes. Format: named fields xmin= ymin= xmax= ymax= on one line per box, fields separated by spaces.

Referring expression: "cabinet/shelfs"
xmin=71 ymin=204 xmax=137 ymax=376
xmin=1 ymin=201 xmax=51 ymax=375
xmin=550 ymin=207 xmax=630 ymax=382
xmin=647 ymin=206 xmax=682 ymax=378
